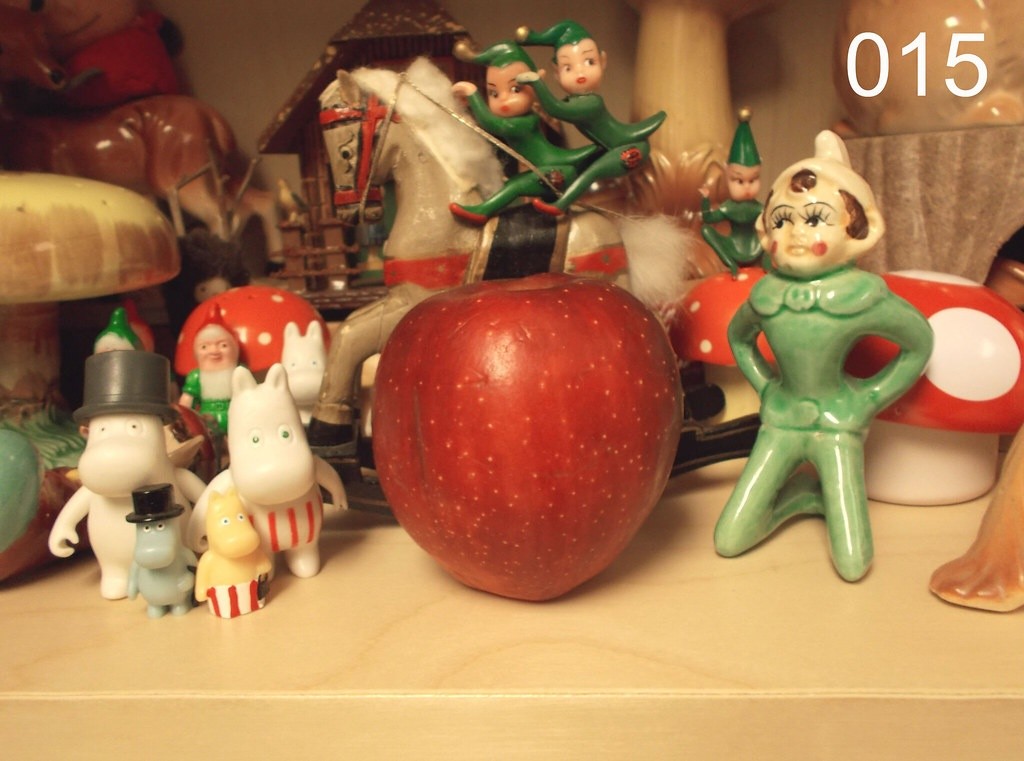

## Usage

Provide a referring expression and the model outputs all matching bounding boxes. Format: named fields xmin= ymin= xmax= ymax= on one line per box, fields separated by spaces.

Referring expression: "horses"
xmin=303 ymin=57 xmax=733 ymax=448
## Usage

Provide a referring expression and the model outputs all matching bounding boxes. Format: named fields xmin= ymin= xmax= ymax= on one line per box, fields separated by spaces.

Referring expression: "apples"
xmin=372 ymin=281 xmax=683 ymax=601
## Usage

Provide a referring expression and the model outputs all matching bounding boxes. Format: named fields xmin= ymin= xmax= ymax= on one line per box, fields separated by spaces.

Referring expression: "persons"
xmin=715 ymin=127 xmax=935 ymax=582
xmin=1 ymin=1 xmax=771 ymax=482
